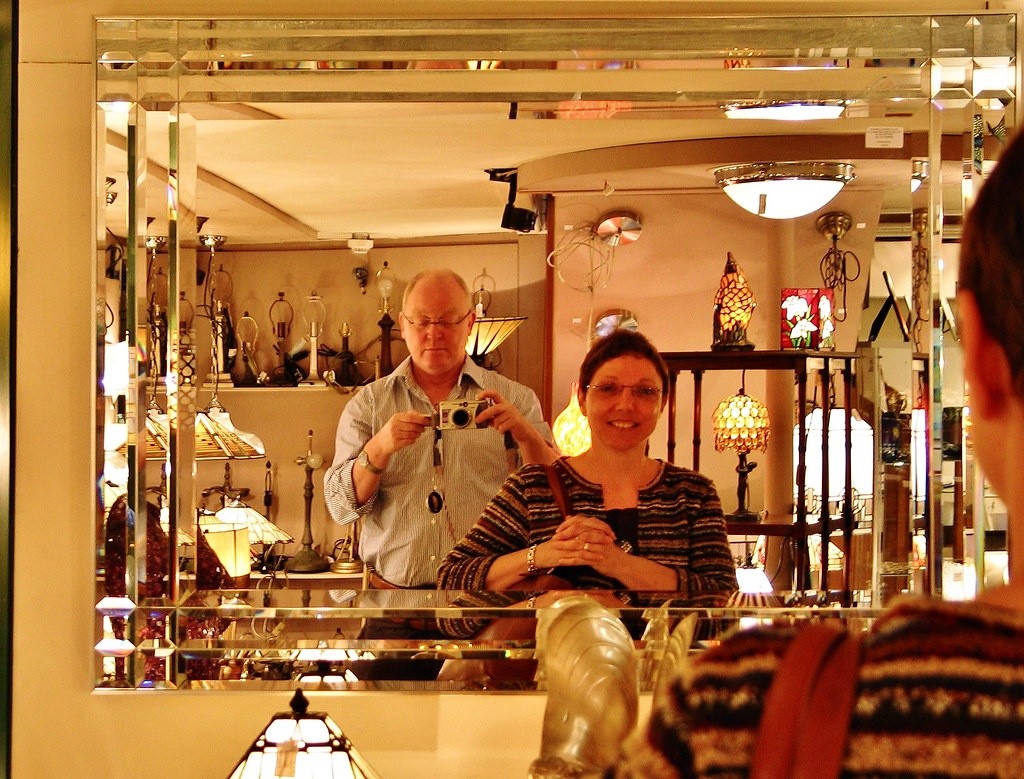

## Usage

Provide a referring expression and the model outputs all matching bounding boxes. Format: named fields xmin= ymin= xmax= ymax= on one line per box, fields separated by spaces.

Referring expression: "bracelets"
xmin=526 ymin=545 xmax=538 ymax=573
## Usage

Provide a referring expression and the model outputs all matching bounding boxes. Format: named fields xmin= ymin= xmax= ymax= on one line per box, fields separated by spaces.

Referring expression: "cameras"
xmin=434 ymin=397 xmax=489 ymax=430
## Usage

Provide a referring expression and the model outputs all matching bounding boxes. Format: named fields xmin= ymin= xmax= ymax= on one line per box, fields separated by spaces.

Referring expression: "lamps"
xmin=710 ymin=388 xmax=771 ymax=521
xmin=711 ymin=251 xmax=759 ymax=352
xmin=709 ymin=162 xmax=856 ymax=220
xmin=119 ymin=259 xmax=399 ymax=574
xmin=781 ymin=212 xmax=859 ymax=354
xmin=551 ymin=382 xmax=594 ymax=460
xmin=464 ymin=293 xmax=530 ymax=370
xmin=729 ymin=554 xmax=783 ymax=610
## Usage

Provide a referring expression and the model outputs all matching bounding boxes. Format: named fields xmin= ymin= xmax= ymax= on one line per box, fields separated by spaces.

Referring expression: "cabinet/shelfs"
xmin=110 ymin=235 xmax=525 ymax=592
xmin=659 ymin=348 xmax=866 ymax=609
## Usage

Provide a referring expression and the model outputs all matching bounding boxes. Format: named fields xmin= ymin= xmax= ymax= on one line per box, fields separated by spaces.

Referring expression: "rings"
xmin=583 ymin=542 xmax=590 ymax=550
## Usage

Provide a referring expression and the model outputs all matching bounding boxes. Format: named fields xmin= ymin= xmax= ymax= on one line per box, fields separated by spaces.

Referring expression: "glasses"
xmin=586 ymin=383 xmax=664 ymax=401
xmin=402 ymin=312 xmax=471 ymax=330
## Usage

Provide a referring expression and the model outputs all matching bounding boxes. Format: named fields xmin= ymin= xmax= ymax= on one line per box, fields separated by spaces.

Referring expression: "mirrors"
xmin=92 ymin=16 xmax=1022 ymax=692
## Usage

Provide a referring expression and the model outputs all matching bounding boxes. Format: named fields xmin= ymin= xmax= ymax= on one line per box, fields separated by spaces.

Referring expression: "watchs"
xmin=356 ymin=450 xmax=385 ymax=475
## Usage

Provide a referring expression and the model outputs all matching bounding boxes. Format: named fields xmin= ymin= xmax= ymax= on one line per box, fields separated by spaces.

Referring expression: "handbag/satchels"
xmin=435 ymin=463 xmax=576 ymax=686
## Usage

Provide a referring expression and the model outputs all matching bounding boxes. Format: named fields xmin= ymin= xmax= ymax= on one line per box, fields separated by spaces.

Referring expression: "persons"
xmin=598 ymin=116 xmax=1024 ymax=778
xmin=436 ymin=327 xmax=739 ymax=642
xmin=322 ymin=268 xmax=562 ymax=681
